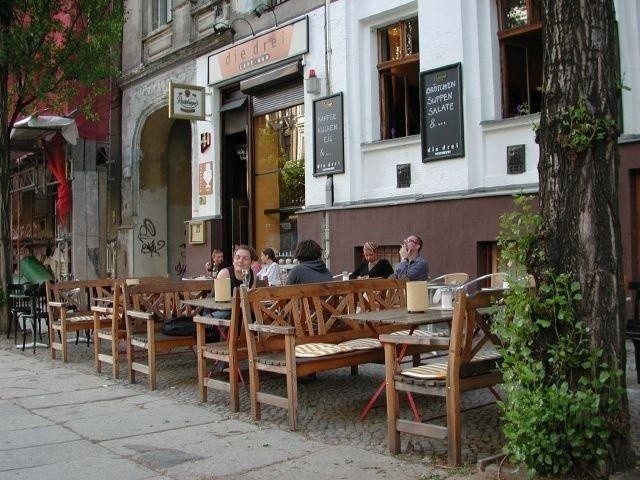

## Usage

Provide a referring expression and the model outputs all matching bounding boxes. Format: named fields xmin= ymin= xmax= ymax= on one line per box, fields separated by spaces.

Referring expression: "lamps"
xmin=210 ymin=2 xmax=279 ymax=46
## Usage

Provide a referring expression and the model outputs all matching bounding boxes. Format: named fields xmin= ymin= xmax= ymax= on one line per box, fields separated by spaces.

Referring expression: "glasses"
xmin=404 ymin=239 xmax=417 ymax=245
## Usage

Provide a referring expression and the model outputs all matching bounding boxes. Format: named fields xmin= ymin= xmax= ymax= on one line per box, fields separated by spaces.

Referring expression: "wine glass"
xmin=207 ymin=265 xmax=212 ymax=279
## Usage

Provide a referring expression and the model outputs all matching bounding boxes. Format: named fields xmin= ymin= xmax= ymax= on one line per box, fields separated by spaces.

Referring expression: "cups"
xmin=441 ymin=291 xmax=453 ymax=309
xmin=279 ymin=268 xmax=287 ymax=286
xmin=406 ymin=281 xmax=429 ymax=313
xmin=213 ymin=278 xmax=232 ymax=302
xmin=503 ymin=282 xmax=510 ymax=290
xmin=343 ymin=271 xmax=349 ymax=280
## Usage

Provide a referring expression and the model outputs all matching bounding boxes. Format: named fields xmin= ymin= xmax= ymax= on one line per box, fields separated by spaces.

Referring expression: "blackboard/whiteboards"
xmin=419 ymin=62 xmax=465 ymax=163
xmin=312 ymin=92 xmax=345 ymax=176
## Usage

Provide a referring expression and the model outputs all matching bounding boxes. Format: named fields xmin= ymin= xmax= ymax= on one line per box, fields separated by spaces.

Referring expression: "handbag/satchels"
xmin=162 ymin=316 xmax=194 ymax=336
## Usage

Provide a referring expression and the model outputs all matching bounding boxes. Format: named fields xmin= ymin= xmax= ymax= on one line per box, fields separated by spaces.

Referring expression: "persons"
xmin=285 ymin=238 xmax=337 ymax=329
xmin=349 ymin=242 xmax=395 ymax=279
xmin=251 ymin=257 xmax=262 ymax=278
xmin=205 ymin=248 xmax=225 ymax=278
xmin=257 ymin=248 xmax=282 ymax=286
xmin=389 ymin=234 xmax=429 ymax=282
xmin=202 ymin=245 xmax=257 ymax=379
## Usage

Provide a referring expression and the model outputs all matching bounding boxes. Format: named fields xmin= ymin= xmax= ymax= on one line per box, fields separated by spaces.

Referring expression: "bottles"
xmin=212 ymin=263 xmax=218 ymax=279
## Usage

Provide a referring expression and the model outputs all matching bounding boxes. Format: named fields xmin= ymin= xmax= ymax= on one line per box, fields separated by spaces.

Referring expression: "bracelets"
xmin=207 ymin=268 xmax=211 ymax=272
